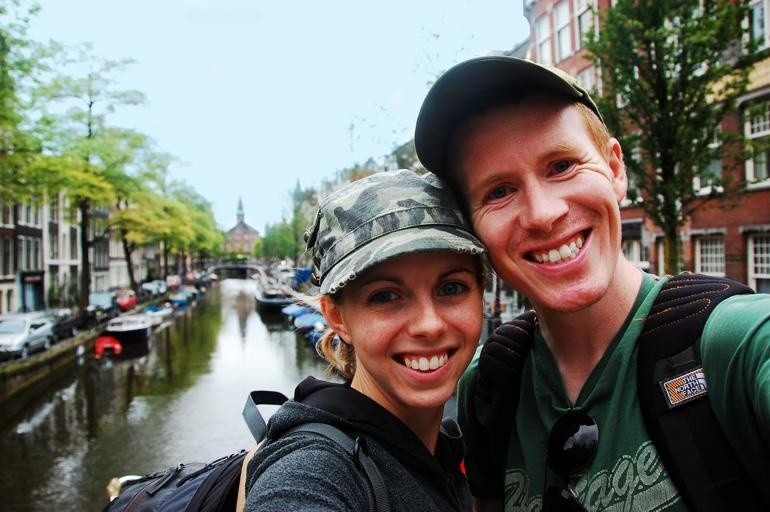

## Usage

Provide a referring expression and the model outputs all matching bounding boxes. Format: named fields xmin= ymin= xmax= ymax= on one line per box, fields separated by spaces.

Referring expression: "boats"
xmin=94 ymin=267 xmax=214 ymax=366
xmin=250 ymin=258 xmax=339 ymax=348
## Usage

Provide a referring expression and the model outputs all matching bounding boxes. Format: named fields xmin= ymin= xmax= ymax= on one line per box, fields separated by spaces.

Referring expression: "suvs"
xmin=0 ymin=289 xmax=119 ymax=364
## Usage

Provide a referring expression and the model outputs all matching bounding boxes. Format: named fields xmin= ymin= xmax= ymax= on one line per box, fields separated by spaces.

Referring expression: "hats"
xmin=304 ymin=169 xmax=488 ymax=295
xmin=415 ymin=56 xmax=607 ymax=175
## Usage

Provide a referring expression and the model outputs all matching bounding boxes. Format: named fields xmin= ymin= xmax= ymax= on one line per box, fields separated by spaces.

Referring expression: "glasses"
xmin=542 ymin=409 xmax=599 ymax=512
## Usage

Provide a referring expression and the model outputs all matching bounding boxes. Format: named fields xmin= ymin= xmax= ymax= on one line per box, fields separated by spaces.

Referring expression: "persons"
xmin=412 ymin=55 xmax=769 ymax=512
xmin=245 ymin=167 xmax=497 ymax=510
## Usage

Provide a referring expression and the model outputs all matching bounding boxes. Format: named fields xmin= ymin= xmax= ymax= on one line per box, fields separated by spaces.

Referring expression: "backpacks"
xmin=104 ymin=391 xmax=390 ymax=512
